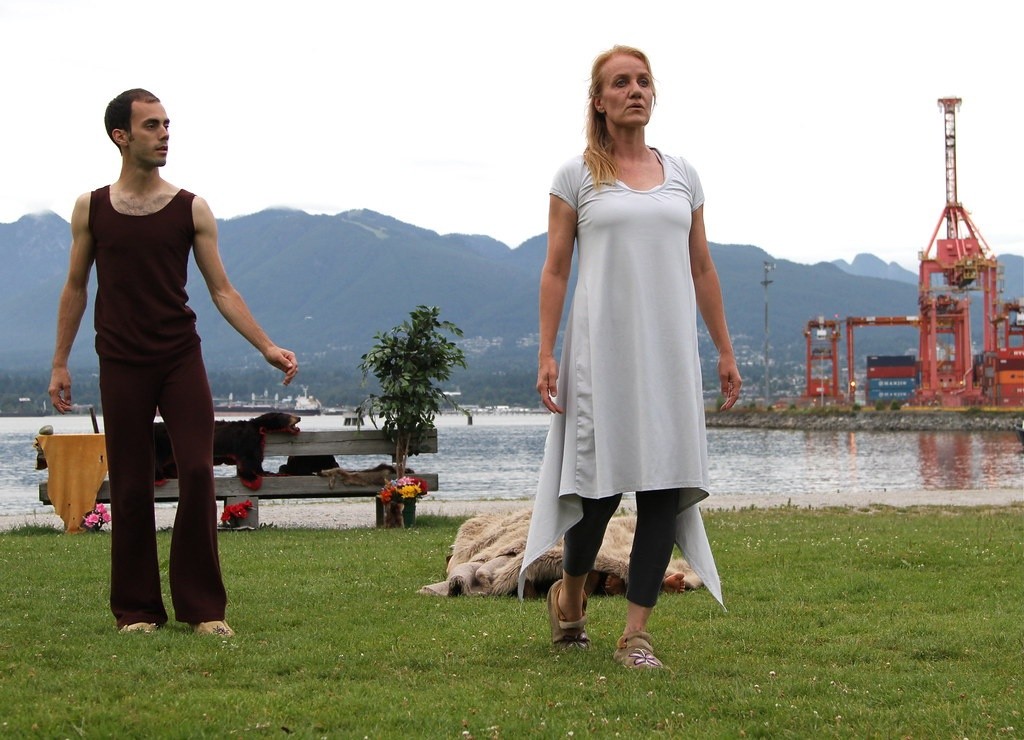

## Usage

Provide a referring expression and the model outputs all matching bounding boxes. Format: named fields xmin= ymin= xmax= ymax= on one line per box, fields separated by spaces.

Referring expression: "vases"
xmin=376 ymin=494 xmax=416 ymax=529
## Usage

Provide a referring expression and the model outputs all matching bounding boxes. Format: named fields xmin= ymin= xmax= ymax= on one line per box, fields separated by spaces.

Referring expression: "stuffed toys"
xmin=151 ymin=412 xmax=300 ymax=490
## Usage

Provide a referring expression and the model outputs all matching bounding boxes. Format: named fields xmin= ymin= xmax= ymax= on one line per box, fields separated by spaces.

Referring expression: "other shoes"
xmin=188 ymin=620 xmax=235 ymax=638
xmin=118 ymin=622 xmax=159 ymax=633
xmin=613 ymin=631 xmax=663 ymax=670
xmin=547 ymin=579 xmax=590 ymax=652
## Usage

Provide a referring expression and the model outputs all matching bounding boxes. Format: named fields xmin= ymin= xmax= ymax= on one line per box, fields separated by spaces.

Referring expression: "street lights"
xmin=759 ymin=261 xmax=776 ymax=409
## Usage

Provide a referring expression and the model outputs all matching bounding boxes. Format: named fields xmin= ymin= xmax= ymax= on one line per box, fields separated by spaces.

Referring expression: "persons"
xmin=522 ymin=573 xmax=686 ymax=599
xmin=537 ymin=46 xmax=741 ymax=670
xmin=49 ymin=88 xmax=297 ymax=637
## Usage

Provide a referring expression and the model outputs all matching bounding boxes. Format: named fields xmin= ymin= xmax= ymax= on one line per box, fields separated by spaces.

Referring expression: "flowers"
xmin=380 ymin=476 xmax=429 ymax=502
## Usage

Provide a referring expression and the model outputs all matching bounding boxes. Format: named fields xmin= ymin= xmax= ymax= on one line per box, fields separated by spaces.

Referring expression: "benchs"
xmin=35 ymin=427 xmax=439 ymax=526
